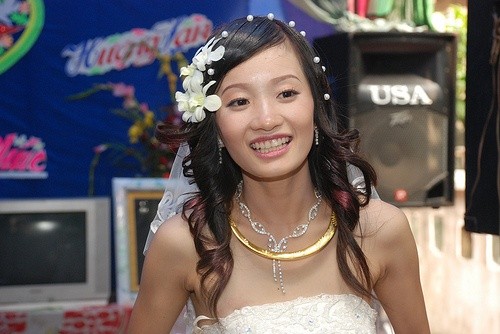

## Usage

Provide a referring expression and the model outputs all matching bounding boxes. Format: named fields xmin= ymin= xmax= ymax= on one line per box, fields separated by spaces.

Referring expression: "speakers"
xmin=311 ymin=32 xmax=455 ymax=209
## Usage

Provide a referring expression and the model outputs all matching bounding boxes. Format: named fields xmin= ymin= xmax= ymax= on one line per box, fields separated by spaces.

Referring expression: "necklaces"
xmin=235 ymin=179 xmax=322 ymax=294
xmin=230 ymin=211 xmax=338 ymax=261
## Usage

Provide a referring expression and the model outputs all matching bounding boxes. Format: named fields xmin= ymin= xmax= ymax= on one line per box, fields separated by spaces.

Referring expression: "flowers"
xmin=175 ymin=36 xmax=226 ymax=122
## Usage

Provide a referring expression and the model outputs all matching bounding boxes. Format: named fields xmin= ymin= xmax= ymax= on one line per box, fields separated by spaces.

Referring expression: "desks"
xmin=0 ymin=304 xmax=132 ymax=334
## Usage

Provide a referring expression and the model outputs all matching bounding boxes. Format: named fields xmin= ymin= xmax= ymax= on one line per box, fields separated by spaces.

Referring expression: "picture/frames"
xmin=112 ymin=177 xmax=168 ymax=306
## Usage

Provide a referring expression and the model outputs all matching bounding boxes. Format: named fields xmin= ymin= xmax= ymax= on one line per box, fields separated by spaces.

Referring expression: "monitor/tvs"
xmin=0 ymin=197 xmax=110 ymax=313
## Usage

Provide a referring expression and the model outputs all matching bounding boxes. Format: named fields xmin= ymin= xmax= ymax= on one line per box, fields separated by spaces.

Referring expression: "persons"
xmin=126 ymin=13 xmax=430 ymax=334
